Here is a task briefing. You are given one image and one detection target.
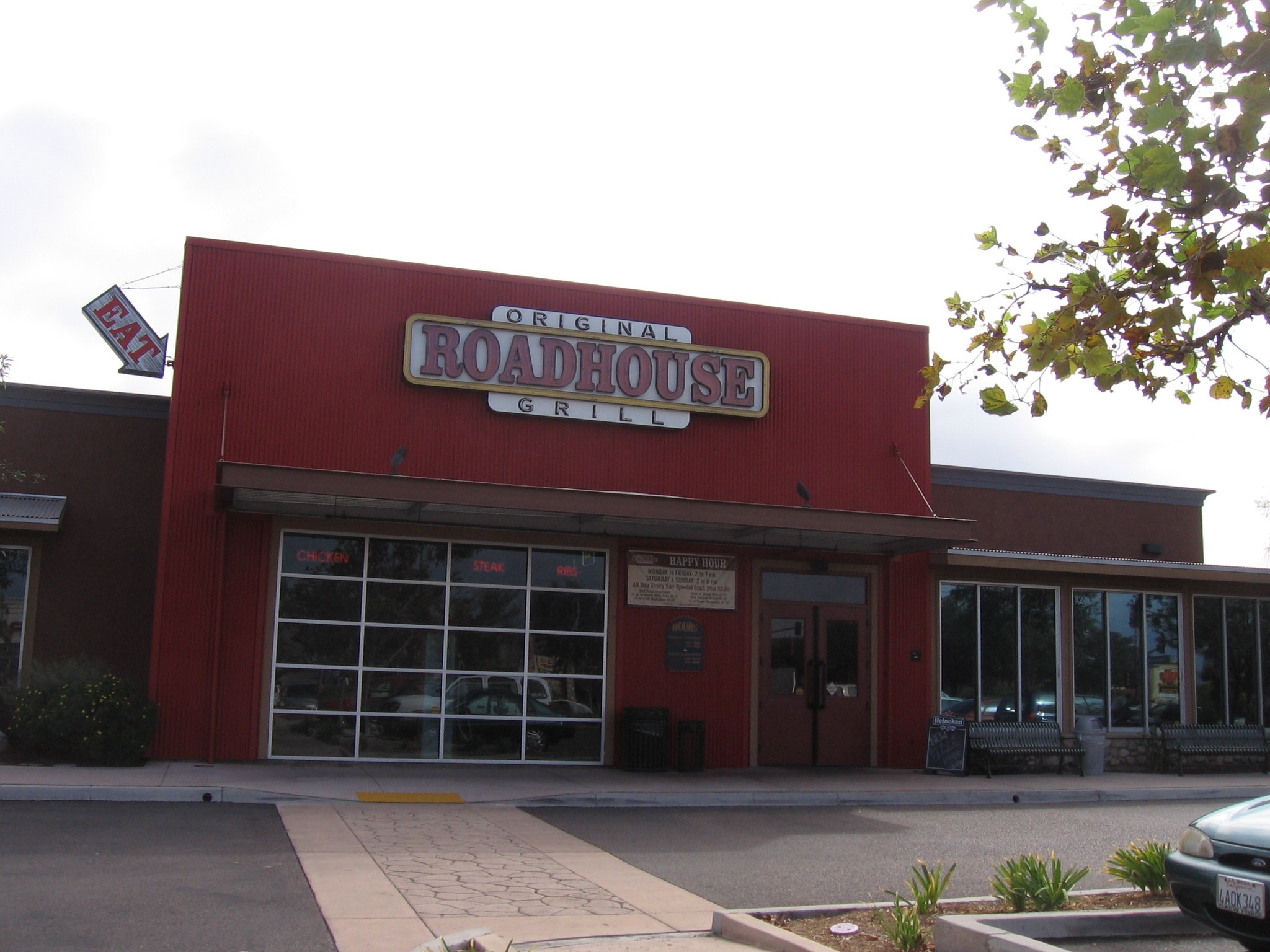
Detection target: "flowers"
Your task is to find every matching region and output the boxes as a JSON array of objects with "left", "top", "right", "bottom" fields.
[{"left": 5, "top": 674, "right": 157, "bottom": 754}]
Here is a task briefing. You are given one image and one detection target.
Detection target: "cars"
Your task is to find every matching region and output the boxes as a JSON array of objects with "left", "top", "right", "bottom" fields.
[
  {"left": 370, "top": 670, "right": 600, "bottom": 753},
  {"left": 939, "top": 687, "right": 1219, "bottom": 727},
  {"left": 828, "top": 683, "right": 856, "bottom": 696},
  {"left": 273, "top": 683, "right": 334, "bottom": 735},
  {"left": 1164, "top": 794, "right": 1270, "bottom": 952}
]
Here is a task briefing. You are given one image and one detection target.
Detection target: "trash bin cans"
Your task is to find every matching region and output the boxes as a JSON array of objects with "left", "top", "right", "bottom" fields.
[
  {"left": 1075, "top": 715, "right": 1108, "bottom": 776},
  {"left": 621, "top": 705, "right": 671, "bottom": 770}
]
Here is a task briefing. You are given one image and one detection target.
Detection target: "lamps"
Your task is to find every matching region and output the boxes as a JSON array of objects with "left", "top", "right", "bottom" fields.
[
  {"left": 796, "top": 481, "right": 811, "bottom": 507},
  {"left": 391, "top": 446, "right": 406, "bottom": 475}
]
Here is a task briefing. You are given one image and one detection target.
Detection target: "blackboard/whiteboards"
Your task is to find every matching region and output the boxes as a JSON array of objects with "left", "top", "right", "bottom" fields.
[{"left": 924, "top": 717, "right": 971, "bottom": 773}]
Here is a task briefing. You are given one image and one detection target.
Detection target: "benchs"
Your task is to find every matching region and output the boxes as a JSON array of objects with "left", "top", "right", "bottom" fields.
[
  {"left": 1159, "top": 725, "right": 1270, "bottom": 776},
  {"left": 970, "top": 722, "right": 1085, "bottom": 781}
]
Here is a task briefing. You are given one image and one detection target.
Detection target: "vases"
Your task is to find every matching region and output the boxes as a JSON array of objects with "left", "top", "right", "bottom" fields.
[
  {"left": 10, "top": 736, "right": 77, "bottom": 767},
  {"left": 77, "top": 746, "right": 150, "bottom": 769}
]
